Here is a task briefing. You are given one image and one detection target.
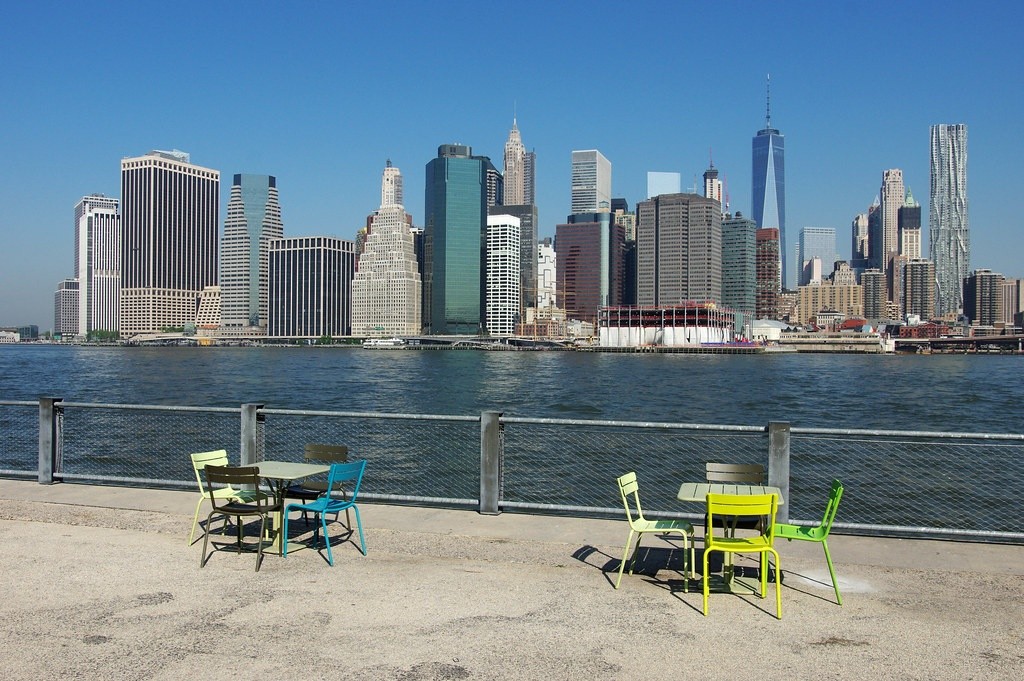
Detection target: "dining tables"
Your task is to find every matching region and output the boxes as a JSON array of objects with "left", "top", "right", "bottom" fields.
[{"left": 763, "top": 477, "right": 844, "bottom": 605}]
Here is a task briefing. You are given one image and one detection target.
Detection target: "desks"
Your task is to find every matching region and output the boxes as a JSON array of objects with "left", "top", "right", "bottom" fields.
[
  {"left": 236, "top": 460, "right": 330, "bottom": 555},
  {"left": 677, "top": 482, "right": 784, "bottom": 595}
]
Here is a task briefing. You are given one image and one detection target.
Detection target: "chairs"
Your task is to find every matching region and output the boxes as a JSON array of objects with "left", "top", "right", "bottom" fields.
[
  {"left": 702, "top": 463, "right": 768, "bottom": 576},
  {"left": 614, "top": 472, "right": 695, "bottom": 593},
  {"left": 283, "top": 459, "right": 367, "bottom": 566},
  {"left": 188, "top": 450, "right": 269, "bottom": 548},
  {"left": 201, "top": 464, "right": 283, "bottom": 572},
  {"left": 282, "top": 444, "right": 351, "bottom": 532},
  {"left": 704, "top": 493, "right": 781, "bottom": 620}
]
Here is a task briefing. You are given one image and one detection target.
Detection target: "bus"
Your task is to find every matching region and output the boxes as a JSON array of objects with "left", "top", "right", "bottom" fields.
[
  {"left": 574, "top": 337, "right": 600, "bottom": 345},
  {"left": 940, "top": 334, "right": 963, "bottom": 339}
]
